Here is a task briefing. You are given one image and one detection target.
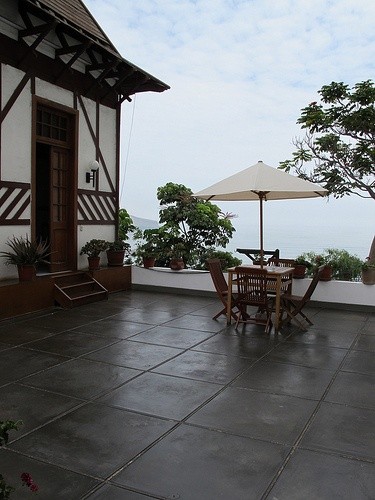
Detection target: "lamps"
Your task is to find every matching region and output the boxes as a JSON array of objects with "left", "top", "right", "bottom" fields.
[{"left": 86, "top": 160, "right": 100, "bottom": 188}]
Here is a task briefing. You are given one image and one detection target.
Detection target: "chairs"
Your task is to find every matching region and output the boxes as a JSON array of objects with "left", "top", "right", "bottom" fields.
[
  {"left": 279, "top": 262, "right": 327, "bottom": 331},
  {"left": 206, "top": 258, "right": 255, "bottom": 322},
  {"left": 253, "top": 258, "right": 295, "bottom": 321},
  {"left": 234, "top": 266, "right": 285, "bottom": 334}
]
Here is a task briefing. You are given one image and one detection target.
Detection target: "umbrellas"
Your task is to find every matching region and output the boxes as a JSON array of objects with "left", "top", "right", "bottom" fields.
[{"left": 190, "top": 161, "right": 329, "bottom": 269}]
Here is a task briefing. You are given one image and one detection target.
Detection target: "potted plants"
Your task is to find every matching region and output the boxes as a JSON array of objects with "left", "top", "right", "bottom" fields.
[
  {"left": 0, "top": 232, "right": 58, "bottom": 282},
  {"left": 292, "top": 248, "right": 375, "bottom": 285},
  {"left": 253, "top": 250, "right": 268, "bottom": 266},
  {"left": 79, "top": 239, "right": 114, "bottom": 269},
  {"left": 137, "top": 249, "right": 160, "bottom": 268},
  {"left": 106, "top": 240, "right": 132, "bottom": 266},
  {"left": 167, "top": 243, "right": 186, "bottom": 271}
]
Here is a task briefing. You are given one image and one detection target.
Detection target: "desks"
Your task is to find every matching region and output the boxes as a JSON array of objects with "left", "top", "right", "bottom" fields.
[{"left": 227, "top": 265, "right": 294, "bottom": 331}]
